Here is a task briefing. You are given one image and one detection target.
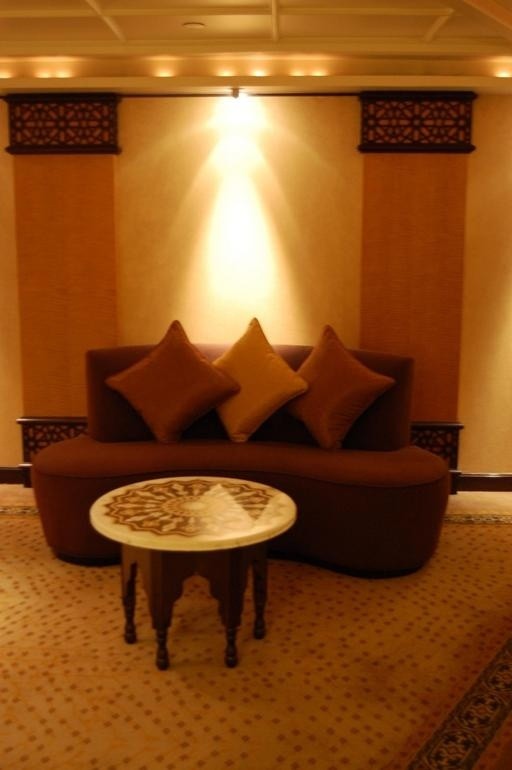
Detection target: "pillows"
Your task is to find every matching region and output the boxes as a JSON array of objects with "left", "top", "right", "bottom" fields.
[
  {"left": 107, "top": 317, "right": 240, "bottom": 444},
  {"left": 295, "top": 324, "right": 396, "bottom": 448},
  {"left": 212, "top": 317, "right": 309, "bottom": 446}
]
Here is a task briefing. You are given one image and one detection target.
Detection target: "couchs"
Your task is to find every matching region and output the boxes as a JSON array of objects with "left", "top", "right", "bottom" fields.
[{"left": 31, "top": 345, "right": 452, "bottom": 580}]
